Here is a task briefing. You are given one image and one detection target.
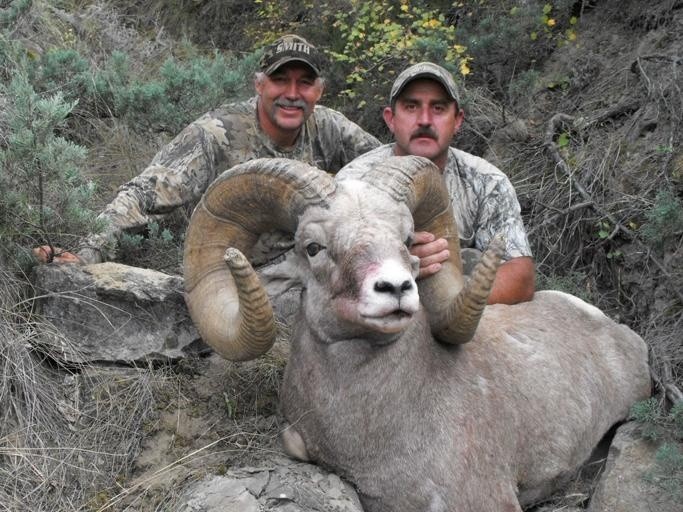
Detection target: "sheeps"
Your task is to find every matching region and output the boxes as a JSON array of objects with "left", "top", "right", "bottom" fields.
[{"left": 181, "top": 155, "right": 653, "bottom": 512}]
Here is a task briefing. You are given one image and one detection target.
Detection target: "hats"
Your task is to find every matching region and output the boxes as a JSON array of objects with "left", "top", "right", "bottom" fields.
[
  {"left": 389, "top": 61, "right": 460, "bottom": 106},
  {"left": 259, "top": 33, "right": 322, "bottom": 80}
]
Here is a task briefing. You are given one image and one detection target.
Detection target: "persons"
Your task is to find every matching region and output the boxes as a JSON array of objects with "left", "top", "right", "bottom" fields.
[
  {"left": 335, "top": 58, "right": 536, "bottom": 305},
  {"left": 33, "top": 33, "right": 382, "bottom": 266}
]
